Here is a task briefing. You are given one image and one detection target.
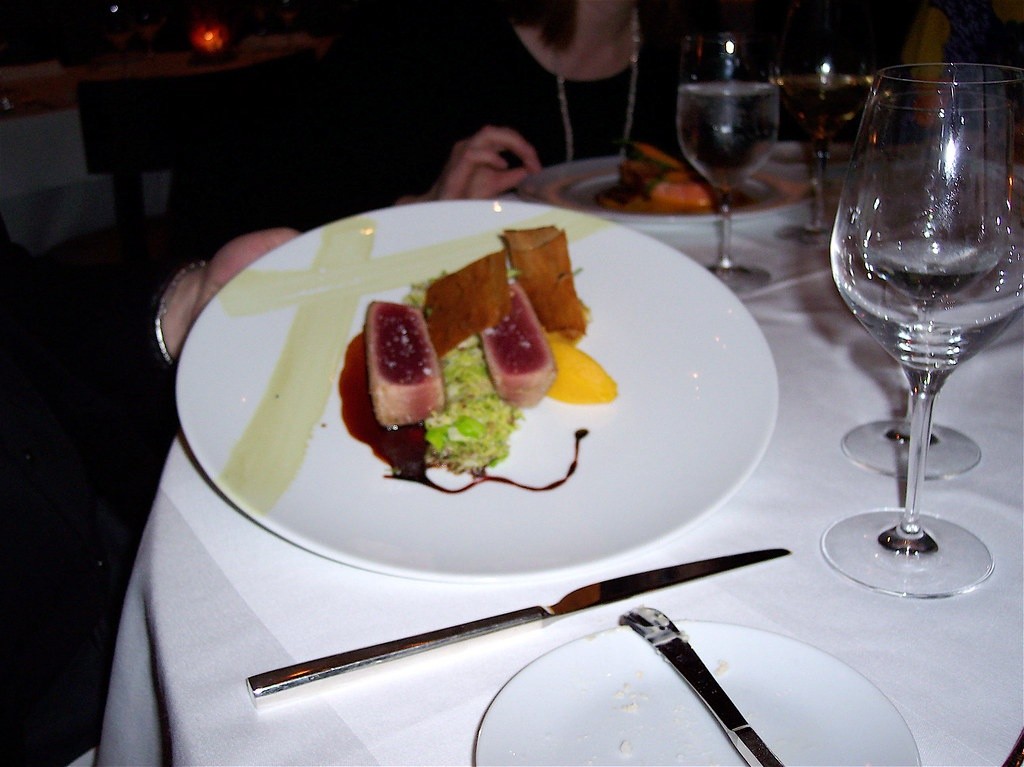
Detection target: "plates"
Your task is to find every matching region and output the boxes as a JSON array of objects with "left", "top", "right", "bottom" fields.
[
  {"left": 474, "top": 618, "right": 922, "bottom": 767},
  {"left": 517, "top": 155, "right": 814, "bottom": 258},
  {"left": 173, "top": 196, "right": 781, "bottom": 584}
]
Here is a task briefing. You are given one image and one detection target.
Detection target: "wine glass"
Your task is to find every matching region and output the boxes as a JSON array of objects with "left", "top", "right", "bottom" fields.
[
  {"left": 840, "top": 95, "right": 1024, "bottom": 482},
  {"left": 827, "top": 57, "right": 1024, "bottom": 601},
  {"left": 677, "top": 32, "right": 780, "bottom": 294}
]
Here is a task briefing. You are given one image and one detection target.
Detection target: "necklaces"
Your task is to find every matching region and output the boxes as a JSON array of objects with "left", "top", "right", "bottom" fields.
[{"left": 550, "top": 10, "right": 638, "bottom": 165}]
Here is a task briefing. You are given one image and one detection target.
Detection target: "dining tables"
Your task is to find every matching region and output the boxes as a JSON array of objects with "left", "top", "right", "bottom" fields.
[{"left": 67, "top": 140, "right": 1024, "bottom": 767}]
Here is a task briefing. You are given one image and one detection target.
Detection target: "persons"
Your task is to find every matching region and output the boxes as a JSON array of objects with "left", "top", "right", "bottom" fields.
[{"left": 0, "top": 0, "right": 1024, "bottom": 766}]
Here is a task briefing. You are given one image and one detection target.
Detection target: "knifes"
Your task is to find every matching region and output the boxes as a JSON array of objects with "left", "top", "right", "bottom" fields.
[{"left": 244, "top": 548, "right": 791, "bottom": 700}]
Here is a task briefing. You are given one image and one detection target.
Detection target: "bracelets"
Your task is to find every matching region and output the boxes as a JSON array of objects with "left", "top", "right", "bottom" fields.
[{"left": 155, "top": 256, "right": 205, "bottom": 371}]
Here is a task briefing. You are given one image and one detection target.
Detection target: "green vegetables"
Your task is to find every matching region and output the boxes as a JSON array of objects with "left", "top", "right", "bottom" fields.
[{"left": 400, "top": 266, "right": 524, "bottom": 471}]
